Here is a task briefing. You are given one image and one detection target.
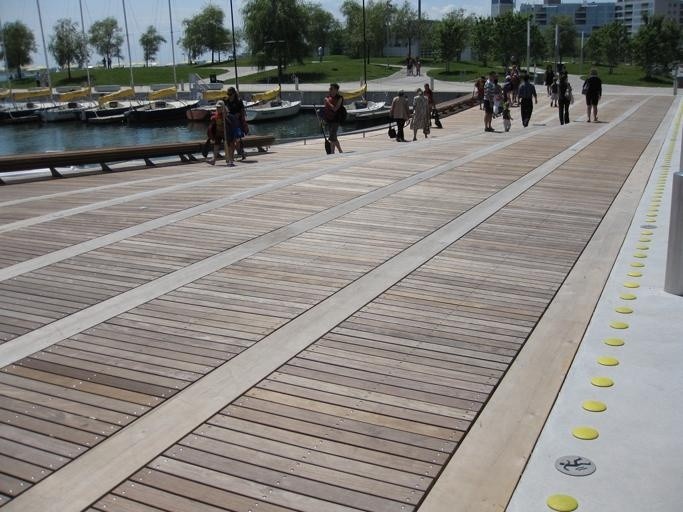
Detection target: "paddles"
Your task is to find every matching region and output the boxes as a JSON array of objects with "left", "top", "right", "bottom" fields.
[
  {"left": 203, "top": 105, "right": 245, "bottom": 164},
  {"left": 312, "top": 102, "right": 331, "bottom": 154}
]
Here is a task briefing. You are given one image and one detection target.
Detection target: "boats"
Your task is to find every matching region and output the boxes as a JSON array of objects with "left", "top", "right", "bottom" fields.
[
  {"left": 0, "top": 61, "right": 158, "bottom": 74},
  {"left": 247, "top": 119, "right": 297, "bottom": 139},
  {"left": 316, "top": 84, "right": 384, "bottom": 121},
  {"left": 123, "top": 87, "right": 199, "bottom": 125},
  {"left": 186, "top": 90, "right": 254, "bottom": 120},
  {"left": 0, "top": 89, "right": 64, "bottom": 121},
  {"left": 33, "top": 86, "right": 99, "bottom": 122},
  {"left": 236, "top": 85, "right": 301, "bottom": 122},
  {"left": 80, "top": 88, "right": 154, "bottom": 120}
]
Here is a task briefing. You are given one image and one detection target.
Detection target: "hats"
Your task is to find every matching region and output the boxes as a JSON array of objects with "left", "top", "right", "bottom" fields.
[{"left": 215, "top": 100, "right": 223, "bottom": 107}]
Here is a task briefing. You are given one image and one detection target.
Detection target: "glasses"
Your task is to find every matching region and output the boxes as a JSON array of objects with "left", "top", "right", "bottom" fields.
[{"left": 227, "top": 93, "right": 232, "bottom": 96}]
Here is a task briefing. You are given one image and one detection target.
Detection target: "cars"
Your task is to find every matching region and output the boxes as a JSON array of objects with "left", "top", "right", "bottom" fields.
[{"left": 669, "top": 64, "right": 683, "bottom": 87}]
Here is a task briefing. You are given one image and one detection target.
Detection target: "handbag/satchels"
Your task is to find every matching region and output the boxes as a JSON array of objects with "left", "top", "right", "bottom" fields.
[{"left": 388, "top": 127, "right": 396, "bottom": 139}]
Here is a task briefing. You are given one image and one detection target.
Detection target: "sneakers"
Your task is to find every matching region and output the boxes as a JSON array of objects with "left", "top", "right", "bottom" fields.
[{"left": 207, "top": 159, "right": 215, "bottom": 165}]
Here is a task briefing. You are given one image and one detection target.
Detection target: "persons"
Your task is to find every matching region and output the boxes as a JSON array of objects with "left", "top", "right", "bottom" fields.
[
  {"left": 107, "top": 56, "right": 112, "bottom": 70},
  {"left": 205, "top": 100, "right": 237, "bottom": 166},
  {"left": 414, "top": 56, "right": 422, "bottom": 77},
  {"left": 405, "top": 52, "right": 415, "bottom": 76},
  {"left": 317, "top": 46, "right": 323, "bottom": 63},
  {"left": 471, "top": 55, "right": 573, "bottom": 132},
  {"left": 582, "top": 69, "right": 602, "bottom": 122},
  {"left": 35, "top": 71, "right": 41, "bottom": 86},
  {"left": 390, "top": 90, "right": 409, "bottom": 142},
  {"left": 323, "top": 82, "right": 344, "bottom": 154},
  {"left": 102, "top": 57, "right": 107, "bottom": 68},
  {"left": 42, "top": 69, "right": 51, "bottom": 87},
  {"left": 410, "top": 87, "right": 431, "bottom": 142},
  {"left": 423, "top": 83, "right": 434, "bottom": 129},
  {"left": 223, "top": 87, "right": 249, "bottom": 162}
]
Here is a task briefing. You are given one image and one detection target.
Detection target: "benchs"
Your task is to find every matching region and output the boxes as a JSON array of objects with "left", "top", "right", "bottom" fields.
[
  {"left": 432, "top": 90, "right": 478, "bottom": 119},
  {"left": 1, "top": 133, "right": 274, "bottom": 183}
]
